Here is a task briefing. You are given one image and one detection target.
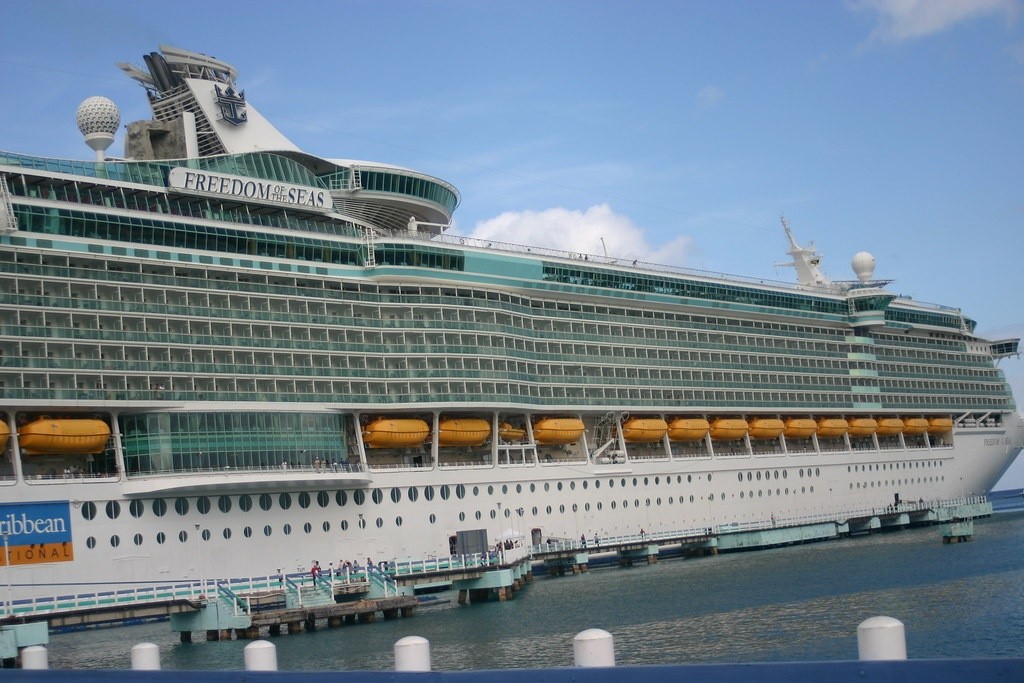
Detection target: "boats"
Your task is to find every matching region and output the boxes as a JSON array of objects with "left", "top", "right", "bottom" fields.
[
  {"left": 362, "top": 419, "right": 429, "bottom": 450},
  {"left": 439, "top": 419, "right": 490, "bottom": 447},
  {"left": 498, "top": 428, "right": 525, "bottom": 440},
  {"left": 621, "top": 417, "right": 953, "bottom": 441},
  {"left": 17, "top": 419, "right": 111, "bottom": 456},
  {"left": 533, "top": 418, "right": 585, "bottom": 444}
]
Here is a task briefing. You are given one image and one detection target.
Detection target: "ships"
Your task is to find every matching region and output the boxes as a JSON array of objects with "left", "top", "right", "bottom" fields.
[{"left": 0, "top": 47, "right": 1024, "bottom": 635}]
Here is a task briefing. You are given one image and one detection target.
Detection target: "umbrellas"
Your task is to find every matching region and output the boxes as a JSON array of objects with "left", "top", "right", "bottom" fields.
[{"left": 495, "top": 528, "right": 526, "bottom": 541}]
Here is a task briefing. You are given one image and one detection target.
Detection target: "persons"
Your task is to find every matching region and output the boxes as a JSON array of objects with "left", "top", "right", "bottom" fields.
[
  {"left": 314, "top": 456, "right": 350, "bottom": 474},
  {"left": 354, "top": 459, "right": 360, "bottom": 470},
  {"left": 337, "top": 559, "right": 360, "bottom": 576},
  {"left": 310, "top": 561, "right": 322, "bottom": 586},
  {"left": 281, "top": 460, "right": 287, "bottom": 473},
  {"left": 329, "top": 561, "right": 334, "bottom": 577},
  {"left": 771, "top": 514, "right": 776, "bottom": 526},
  {"left": 851, "top": 437, "right": 871, "bottom": 450},
  {"left": 494, "top": 540, "right": 524, "bottom": 558},
  {"left": 63, "top": 467, "right": 70, "bottom": 479},
  {"left": 581, "top": 534, "right": 586, "bottom": 548},
  {"left": 366, "top": 557, "right": 373, "bottom": 573},
  {"left": 640, "top": 528, "right": 646, "bottom": 541},
  {"left": 75, "top": 467, "right": 83, "bottom": 478},
  {"left": 919, "top": 496, "right": 923, "bottom": 509},
  {"left": 594, "top": 533, "right": 600, "bottom": 547}
]
